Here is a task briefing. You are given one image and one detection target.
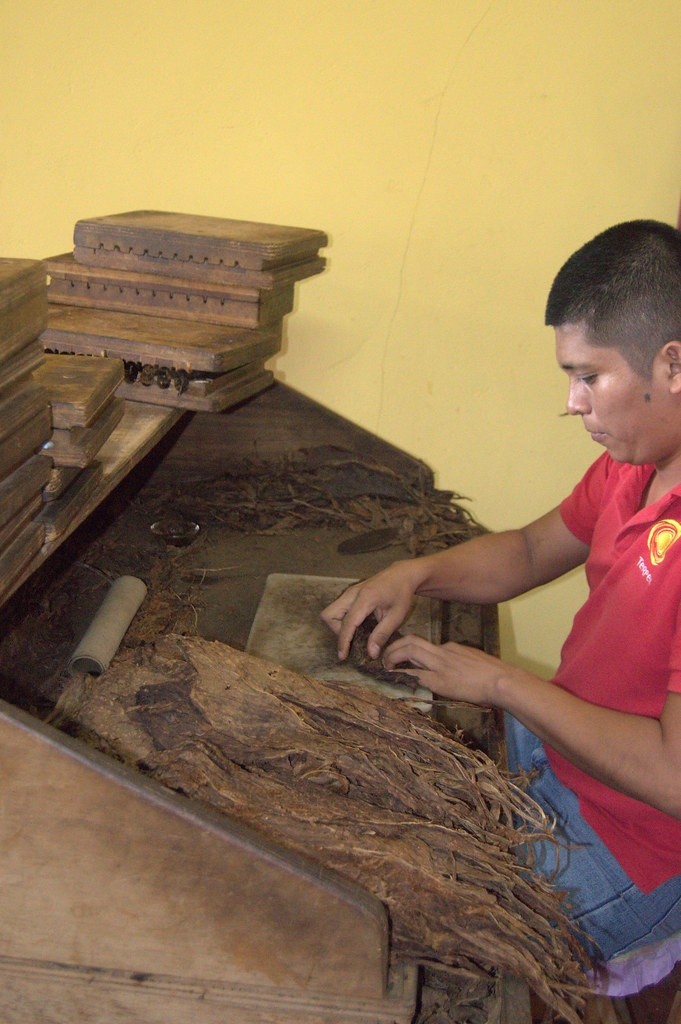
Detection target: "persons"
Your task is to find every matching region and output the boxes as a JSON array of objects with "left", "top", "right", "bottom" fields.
[{"left": 321, "top": 220, "right": 681, "bottom": 973}]
